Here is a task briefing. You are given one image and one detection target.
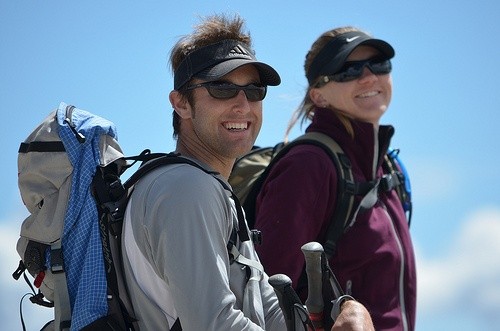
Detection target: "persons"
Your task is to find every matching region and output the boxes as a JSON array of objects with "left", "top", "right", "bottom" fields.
[
  {"left": 117, "top": 9, "right": 377, "bottom": 331},
  {"left": 250, "top": 24, "right": 418, "bottom": 331}
]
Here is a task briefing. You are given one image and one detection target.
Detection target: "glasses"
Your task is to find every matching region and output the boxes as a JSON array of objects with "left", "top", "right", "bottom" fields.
[
  {"left": 188, "top": 79, "right": 267, "bottom": 102},
  {"left": 311, "top": 54, "right": 392, "bottom": 89}
]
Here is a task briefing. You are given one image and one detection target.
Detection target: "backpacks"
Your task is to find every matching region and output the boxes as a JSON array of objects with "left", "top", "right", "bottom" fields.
[
  {"left": 227, "top": 131, "right": 414, "bottom": 300},
  {"left": 12, "top": 100, "right": 266, "bottom": 331}
]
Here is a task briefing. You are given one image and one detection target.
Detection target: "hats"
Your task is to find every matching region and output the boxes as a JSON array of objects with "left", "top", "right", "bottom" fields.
[
  {"left": 305, "top": 30, "right": 395, "bottom": 86},
  {"left": 173, "top": 38, "right": 281, "bottom": 90}
]
